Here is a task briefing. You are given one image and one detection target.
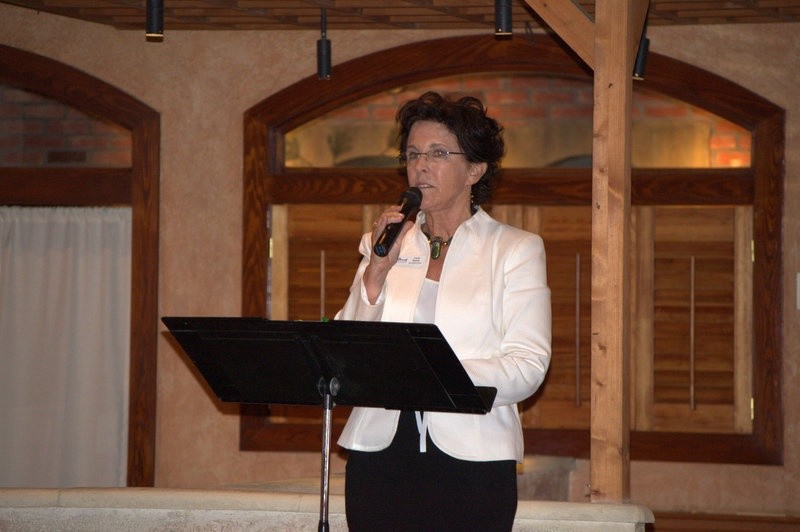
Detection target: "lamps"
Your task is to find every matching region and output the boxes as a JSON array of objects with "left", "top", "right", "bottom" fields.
[
  {"left": 146, "top": 0, "right": 163, "bottom": 41},
  {"left": 493, "top": 0, "right": 512, "bottom": 36},
  {"left": 632, "top": 0, "right": 651, "bottom": 80},
  {"left": 317, "top": 8, "right": 331, "bottom": 80}
]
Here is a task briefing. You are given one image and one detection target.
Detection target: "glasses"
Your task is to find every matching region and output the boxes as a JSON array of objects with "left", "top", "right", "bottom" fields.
[{"left": 400, "top": 145, "right": 467, "bottom": 163}]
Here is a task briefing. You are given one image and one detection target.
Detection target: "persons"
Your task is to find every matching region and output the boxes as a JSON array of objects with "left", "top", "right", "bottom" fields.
[{"left": 333, "top": 91, "right": 551, "bottom": 532}]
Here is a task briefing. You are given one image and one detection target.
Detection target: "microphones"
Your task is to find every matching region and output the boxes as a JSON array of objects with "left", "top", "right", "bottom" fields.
[{"left": 373, "top": 186, "right": 424, "bottom": 258}]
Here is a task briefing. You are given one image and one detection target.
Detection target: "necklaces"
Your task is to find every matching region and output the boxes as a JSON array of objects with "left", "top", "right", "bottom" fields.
[{"left": 422, "top": 229, "right": 454, "bottom": 259}]
